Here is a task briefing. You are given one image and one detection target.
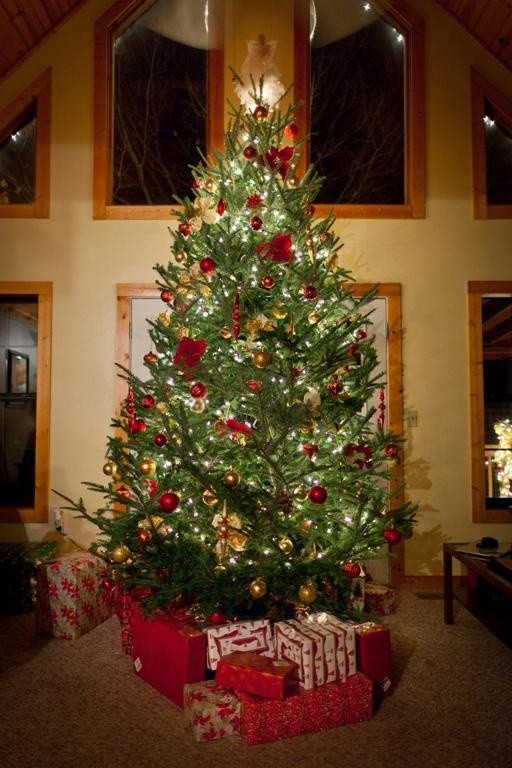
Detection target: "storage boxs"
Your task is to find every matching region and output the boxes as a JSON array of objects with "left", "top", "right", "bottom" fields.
[
  {"left": 36, "top": 550, "right": 115, "bottom": 641},
  {"left": 120, "top": 581, "right": 395, "bottom": 746}
]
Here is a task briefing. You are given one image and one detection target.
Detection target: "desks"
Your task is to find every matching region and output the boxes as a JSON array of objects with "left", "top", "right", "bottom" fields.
[{"left": 442, "top": 541, "right": 512, "bottom": 653}]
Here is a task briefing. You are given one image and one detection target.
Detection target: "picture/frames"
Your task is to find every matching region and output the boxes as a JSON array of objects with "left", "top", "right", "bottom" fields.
[{"left": 5, "top": 349, "right": 29, "bottom": 408}]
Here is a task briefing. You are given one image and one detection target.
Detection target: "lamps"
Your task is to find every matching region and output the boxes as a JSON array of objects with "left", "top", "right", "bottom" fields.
[{"left": 235, "top": 32, "right": 287, "bottom": 115}]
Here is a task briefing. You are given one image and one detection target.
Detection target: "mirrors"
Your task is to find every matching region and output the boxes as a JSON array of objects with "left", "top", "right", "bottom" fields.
[{"left": 0, "top": 281, "right": 53, "bottom": 525}]
